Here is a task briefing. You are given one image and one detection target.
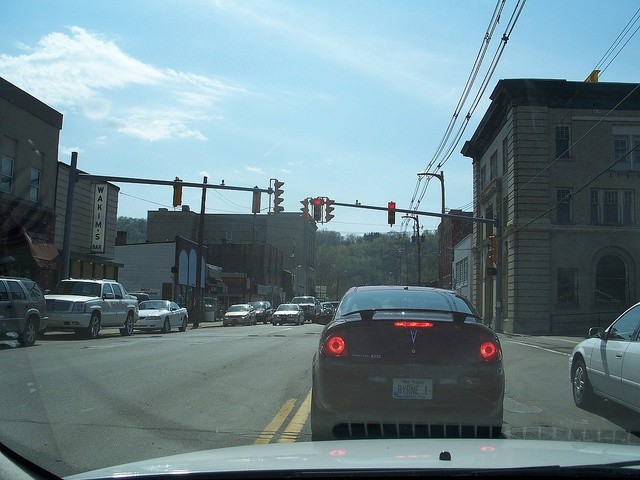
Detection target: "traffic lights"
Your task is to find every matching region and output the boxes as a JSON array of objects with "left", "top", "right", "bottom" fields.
[
  {"left": 323, "top": 197, "right": 334, "bottom": 223},
  {"left": 310, "top": 199, "right": 323, "bottom": 222},
  {"left": 489, "top": 236, "right": 498, "bottom": 263},
  {"left": 269, "top": 179, "right": 284, "bottom": 215},
  {"left": 300, "top": 197, "right": 312, "bottom": 223},
  {"left": 387, "top": 202, "right": 395, "bottom": 224}
]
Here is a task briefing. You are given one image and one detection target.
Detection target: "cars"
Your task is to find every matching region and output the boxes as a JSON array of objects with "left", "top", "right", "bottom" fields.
[
  {"left": 569, "top": 303, "right": 639, "bottom": 412},
  {"left": 317, "top": 302, "right": 335, "bottom": 323},
  {"left": 272, "top": 303, "right": 304, "bottom": 325},
  {"left": 248, "top": 301, "right": 273, "bottom": 324},
  {"left": 133, "top": 300, "right": 188, "bottom": 334},
  {"left": 224, "top": 303, "right": 256, "bottom": 325},
  {"left": 313, "top": 286, "right": 504, "bottom": 434}
]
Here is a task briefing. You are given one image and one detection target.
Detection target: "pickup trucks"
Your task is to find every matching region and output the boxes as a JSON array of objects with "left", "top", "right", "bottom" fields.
[{"left": 44, "top": 280, "right": 138, "bottom": 338}]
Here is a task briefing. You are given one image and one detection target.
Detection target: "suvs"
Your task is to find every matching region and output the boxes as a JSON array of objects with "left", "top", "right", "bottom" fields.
[
  {"left": 129, "top": 291, "right": 149, "bottom": 304},
  {"left": 0, "top": 276, "right": 48, "bottom": 347},
  {"left": 291, "top": 296, "right": 321, "bottom": 323}
]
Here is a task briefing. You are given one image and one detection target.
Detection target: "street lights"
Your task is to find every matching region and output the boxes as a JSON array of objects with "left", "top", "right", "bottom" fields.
[
  {"left": 418, "top": 172, "right": 445, "bottom": 289},
  {"left": 402, "top": 216, "right": 421, "bottom": 285}
]
